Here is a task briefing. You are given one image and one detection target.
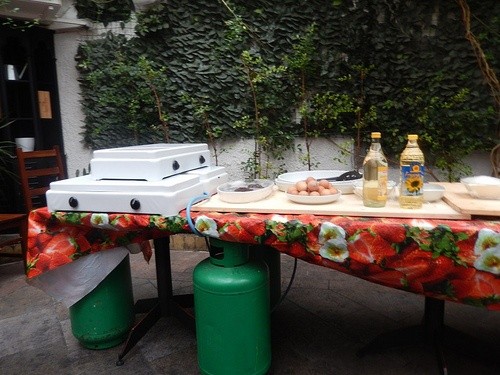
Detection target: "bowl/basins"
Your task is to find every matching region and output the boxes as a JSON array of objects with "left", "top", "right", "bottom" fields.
[
  {"left": 460, "top": 175, "right": 500, "bottom": 200},
  {"left": 15, "top": 137, "right": 35, "bottom": 152},
  {"left": 351, "top": 179, "right": 396, "bottom": 200},
  {"left": 422, "top": 183, "right": 446, "bottom": 202}
]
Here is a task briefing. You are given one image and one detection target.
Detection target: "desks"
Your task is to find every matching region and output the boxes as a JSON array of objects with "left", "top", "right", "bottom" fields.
[{"left": 27, "top": 179, "right": 500, "bottom": 375}]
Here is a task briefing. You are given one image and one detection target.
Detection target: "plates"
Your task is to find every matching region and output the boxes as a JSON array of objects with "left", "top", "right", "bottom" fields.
[
  {"left": 285, "top": 191, "right": 341, "bottom": 204},
  {"left": 217, "top": 179, "right": 274, "bottom": 204},
  {"left": 275, "top": 170, "right": 364, "bottom": 195}
]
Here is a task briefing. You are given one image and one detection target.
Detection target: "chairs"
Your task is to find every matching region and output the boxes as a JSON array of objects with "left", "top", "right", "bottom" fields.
[
  {"left": 15, "top": 144, "right": 66, "bottom": 213},
  {"left": 0, "top": 213, "right": 29, "bottom": 273}
]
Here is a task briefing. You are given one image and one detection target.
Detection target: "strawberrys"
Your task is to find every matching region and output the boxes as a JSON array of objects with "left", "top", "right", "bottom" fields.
[{"left": 26, "top": 209, "right": 500, "bottom": 309}]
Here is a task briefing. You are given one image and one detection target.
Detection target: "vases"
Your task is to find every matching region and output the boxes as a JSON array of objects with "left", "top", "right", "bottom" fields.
[{"left": 15, "top": 137, "right": 35, "bottom": 153}]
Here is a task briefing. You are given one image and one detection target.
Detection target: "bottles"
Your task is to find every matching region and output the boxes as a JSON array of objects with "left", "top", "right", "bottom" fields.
[
  {"left": 363, "top": 132, "right": 388, "bottom": 208},
  {"left": 398, "top": 135, "right": 424, "bottom": 209},
  {"left": 216, "top": 177, "right": 223, "bottom": 194}
]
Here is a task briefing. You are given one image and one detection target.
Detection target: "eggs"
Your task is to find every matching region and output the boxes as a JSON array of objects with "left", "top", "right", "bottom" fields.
[{"left": 287, "top": 177, "right": 337, "bottom": 196}]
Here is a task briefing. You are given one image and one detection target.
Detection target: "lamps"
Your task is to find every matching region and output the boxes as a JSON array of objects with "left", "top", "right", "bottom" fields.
[{"left": 4, "top": 62, "right": 28, "bottom": 81}]
